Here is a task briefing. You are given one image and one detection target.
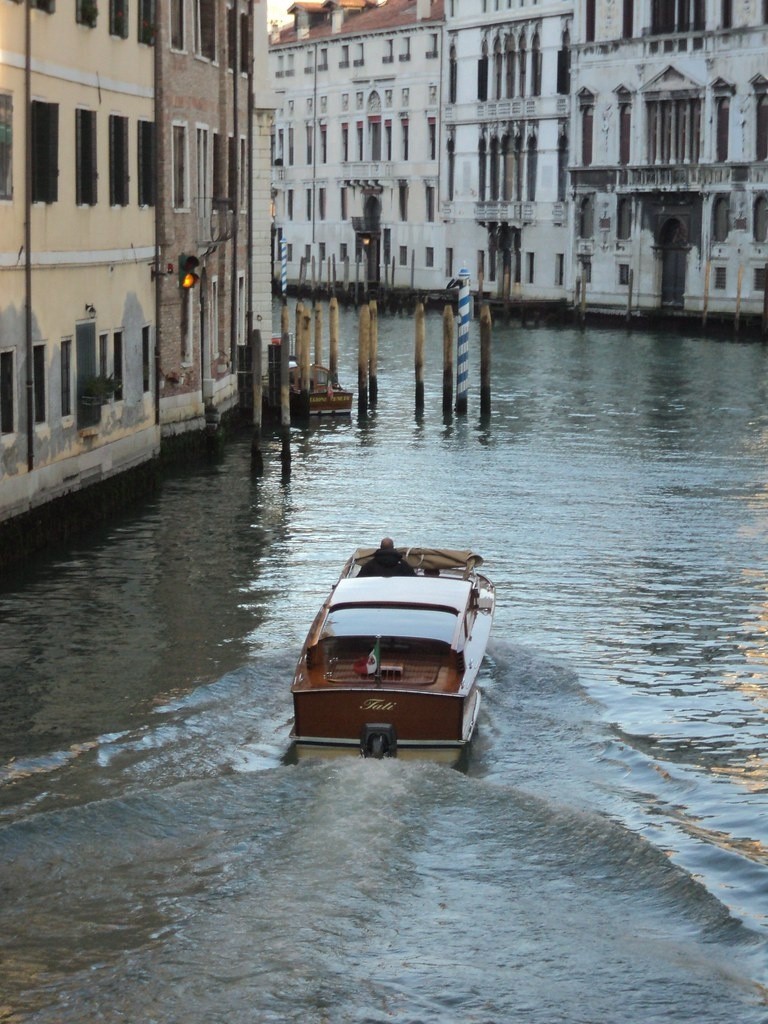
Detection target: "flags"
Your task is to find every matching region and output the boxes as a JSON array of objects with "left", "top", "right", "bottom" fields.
[{"left": 354, "top": 642, "right": 378, "bottom": 674}]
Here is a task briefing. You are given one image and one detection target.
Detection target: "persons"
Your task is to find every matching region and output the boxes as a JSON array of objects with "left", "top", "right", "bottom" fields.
[{"left": 356, "top": 538, "right": 418, "bottom": 577}]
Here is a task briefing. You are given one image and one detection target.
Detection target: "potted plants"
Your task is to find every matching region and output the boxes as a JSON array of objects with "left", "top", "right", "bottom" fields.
[{"left": 80, "top": 372, "right": 123, "bottom": 406}]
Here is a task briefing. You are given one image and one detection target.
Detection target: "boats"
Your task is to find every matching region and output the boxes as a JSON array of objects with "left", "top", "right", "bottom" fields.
[
  {"left": 286, "top": 361, "right": 353, "bottom": 415},
  {"left": 287, "top": 546, "right": 497, "bottom": 770}
]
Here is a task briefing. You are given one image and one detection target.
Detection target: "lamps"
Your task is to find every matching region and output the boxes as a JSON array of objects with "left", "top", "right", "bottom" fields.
[{"left": 85, "top": 303, "right": 96, "bottom": 319}]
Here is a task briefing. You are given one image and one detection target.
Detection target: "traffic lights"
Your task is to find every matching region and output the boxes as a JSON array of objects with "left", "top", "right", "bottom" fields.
[{"left": 178, "top": 254, "right": 201, "bottom": 290}]
